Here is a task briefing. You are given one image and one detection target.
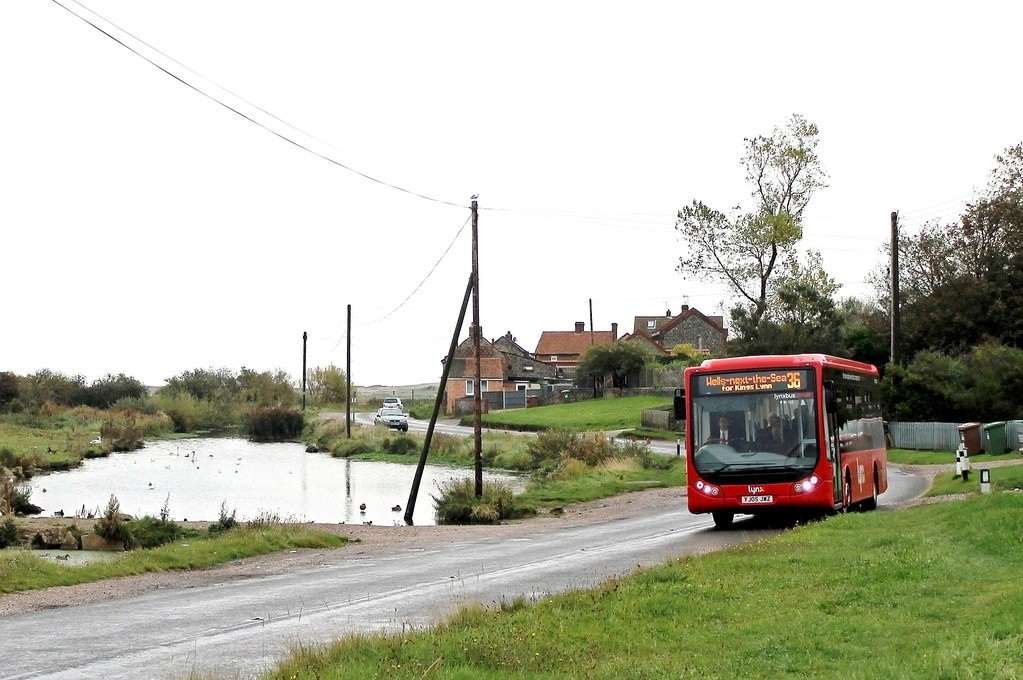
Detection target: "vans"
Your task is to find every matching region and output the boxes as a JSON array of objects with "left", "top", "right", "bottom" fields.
[{"left": 382, "top": 398, "right": 402, "bottom": 409}]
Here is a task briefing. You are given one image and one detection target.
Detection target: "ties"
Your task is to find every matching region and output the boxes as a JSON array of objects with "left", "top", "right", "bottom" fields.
[{"left": 722, "top": 432, "right": 725, "bottom": 444}]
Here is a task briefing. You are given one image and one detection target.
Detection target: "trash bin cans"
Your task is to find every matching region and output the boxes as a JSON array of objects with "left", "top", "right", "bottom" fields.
[
  {"left": 982, "top": 420, "right": 1011, "bottom": 457},
  {"left": 957, "top": 422, "right": 982, "bottom": 458}
]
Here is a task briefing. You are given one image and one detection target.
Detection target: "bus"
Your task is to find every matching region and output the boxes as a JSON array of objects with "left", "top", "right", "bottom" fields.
[{"left": 671, "top": 352, "right": 890, "bottom": 530}]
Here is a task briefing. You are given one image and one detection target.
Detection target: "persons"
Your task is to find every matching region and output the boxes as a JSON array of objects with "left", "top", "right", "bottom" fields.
[
  {"left": 707, "top": 416, "right": 735, "bottom": 446},
  {"left": 754, "top": 405, "right": 813, "bottom": 449}
]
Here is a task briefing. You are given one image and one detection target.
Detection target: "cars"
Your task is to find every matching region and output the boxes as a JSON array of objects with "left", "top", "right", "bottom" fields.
[{"left": 374, "top": 407, "right": 409, "bottom": 432}]
